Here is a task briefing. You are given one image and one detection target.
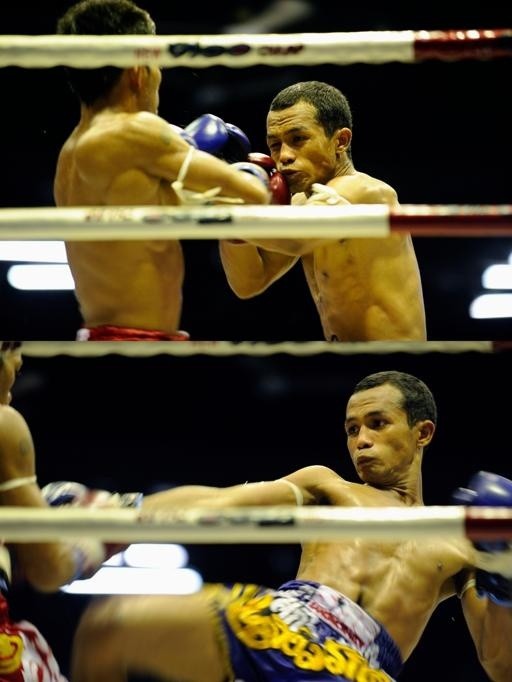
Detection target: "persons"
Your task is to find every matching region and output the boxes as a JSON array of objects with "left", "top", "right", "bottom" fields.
[
  {"left": 41, "top": 372, "right": 512, "bottom": 682},
  {"left": 54, "top": 1, "right": 269, "bottom": 341},
  {"left": 219, "top": 81, "right": 428, "bottom": 340},
  {"left": 0, "top": 341, "right": 131, "bottom": 682}
]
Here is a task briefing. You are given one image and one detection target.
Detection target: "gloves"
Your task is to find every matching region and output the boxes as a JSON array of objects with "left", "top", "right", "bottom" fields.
[
  {"left": 42, "top": 481, "right": 143, "bottom": 586},
  {"left": 171, "top": 113, "right": 252, "bottom": 162},
  {"left": 453, "top": 468, "right": 512, "bottom": 609},
  {"left": 231, "top": 152, "right": 290, "bottom": 206}
]
